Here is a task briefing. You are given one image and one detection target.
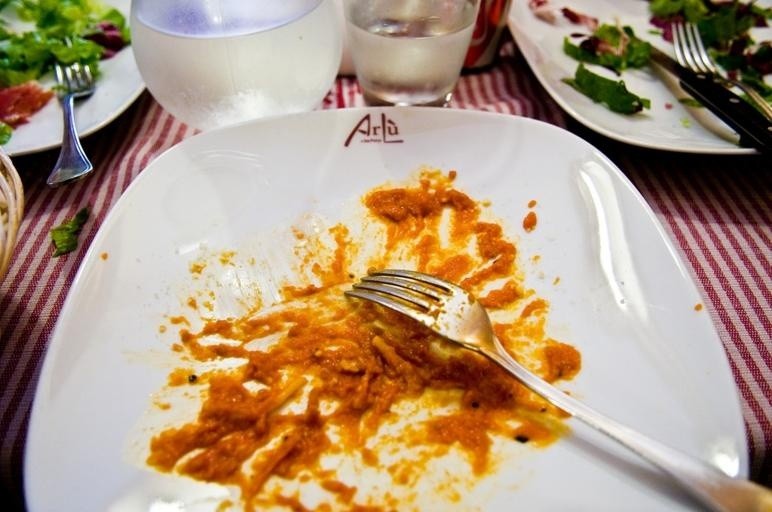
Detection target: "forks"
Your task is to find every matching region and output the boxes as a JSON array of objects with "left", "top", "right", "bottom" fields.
[
  {"left": 672, "top": 16, "right": 772, "bottom": 124},
  {"left": 342, "top": 266, "right": 771, "bottom": 509},
  {"left": 44, "top": 61, "right": 102, "bottom": 185}
]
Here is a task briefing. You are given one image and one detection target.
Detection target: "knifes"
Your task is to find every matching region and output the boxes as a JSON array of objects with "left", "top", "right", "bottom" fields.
[{"left": 571, "top": 10, "right": 770, "bottom": 157}]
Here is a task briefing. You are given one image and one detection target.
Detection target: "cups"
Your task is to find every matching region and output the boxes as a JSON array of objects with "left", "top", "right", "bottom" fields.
[
  {"left": 344, "top": 2, "right": 480, "bottom": 108},
  {"left": 133, "top": 0, "right": 346, "bottom": 125}
]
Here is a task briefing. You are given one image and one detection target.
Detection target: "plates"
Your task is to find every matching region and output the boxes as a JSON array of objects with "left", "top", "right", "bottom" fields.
[
  {"left": 508, "top": 1, "right": 763, "bottom": 155},
  {"left": 0, "top": 0, "right": 146, "bottom": 154},
  {"left": 21, "top": 104, "right": 751, "bottom": 505}
]
